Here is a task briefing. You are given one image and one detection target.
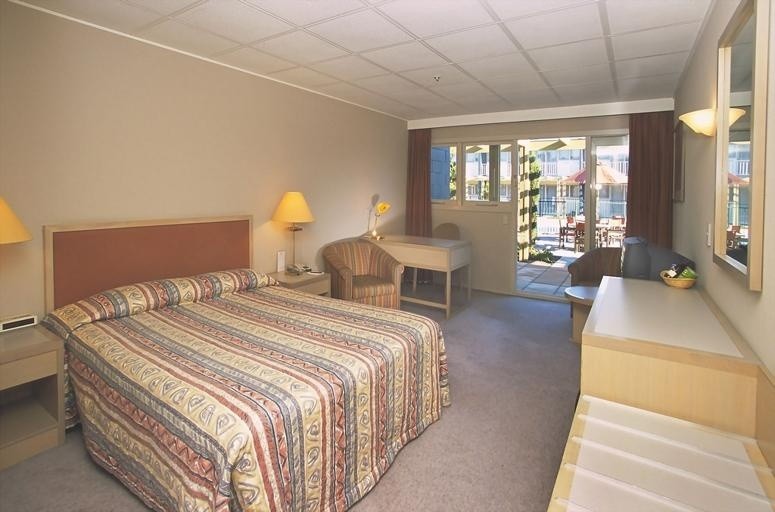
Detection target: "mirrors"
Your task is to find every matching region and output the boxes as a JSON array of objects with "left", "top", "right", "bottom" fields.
[{"left": 713, "top": 0, "right": 771, "bottom": 295}]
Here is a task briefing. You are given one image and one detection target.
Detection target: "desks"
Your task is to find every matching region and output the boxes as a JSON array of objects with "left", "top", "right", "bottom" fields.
[{"left": 379, "top": 233, "right": 471, "bottom": 316}]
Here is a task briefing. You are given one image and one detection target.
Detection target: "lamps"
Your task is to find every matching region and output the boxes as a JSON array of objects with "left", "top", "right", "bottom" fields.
[
  {"left": 0, "top": 199, "right": 34, "bottom": 244},
  {"left": 272, "top": 190, "right": 314, "bottom": 272},
  {"left": 676, "top": 105, "right": 713, "bottom": 136},
  {"left": 729, "top": 106, "right": 747, "bottom": 132},
  {"left": 370, "top": 202, "right": 390, "bottom": 236}
]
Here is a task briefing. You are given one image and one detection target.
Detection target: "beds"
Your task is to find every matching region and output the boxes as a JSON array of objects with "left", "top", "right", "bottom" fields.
[{"left": 40, "top": 216, "right": 452, "bottom": 512}]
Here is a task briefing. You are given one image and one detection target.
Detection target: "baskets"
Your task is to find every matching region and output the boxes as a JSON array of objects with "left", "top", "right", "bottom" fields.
[{"left": 659, "top": 269, "right": 697, "bottom": 289}]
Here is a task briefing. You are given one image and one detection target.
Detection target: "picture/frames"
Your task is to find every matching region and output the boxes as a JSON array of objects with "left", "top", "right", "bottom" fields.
[{"left": 672, "top": 115, "right": 686, "bottom": 202}]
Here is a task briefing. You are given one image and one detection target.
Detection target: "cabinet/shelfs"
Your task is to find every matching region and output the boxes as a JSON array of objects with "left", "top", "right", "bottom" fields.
[
  {"left": 578, "top": 277, "right": 759, "bottom": 432},
  {"left": 0, "top": 322, "right": 64, "bottom": 472},
  {"left": 266, "top": 268, "right": 330, "bottom": 298},
  {"left": 550, "top": 395, "right": 774, "bottom": 512}
]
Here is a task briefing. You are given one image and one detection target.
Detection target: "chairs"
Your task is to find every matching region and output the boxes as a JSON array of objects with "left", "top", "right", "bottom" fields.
[
  {"left": 322, "top": 237, "right": 403, "bottom": 310},
  {"left": 430, "top": 225, "right": 461, "bottom": 294},
  {"left": 557, "top": 207, "right": 626, "bottom": 252}
]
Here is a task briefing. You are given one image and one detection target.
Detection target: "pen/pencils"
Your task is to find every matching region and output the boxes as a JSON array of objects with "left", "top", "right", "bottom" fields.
[{"left": 311, "top": 272, "right": 322, "bottom": 273}]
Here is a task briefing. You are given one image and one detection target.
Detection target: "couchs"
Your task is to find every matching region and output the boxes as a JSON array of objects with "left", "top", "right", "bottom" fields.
[{"left": 567, "top": 245, "right": 697, "bottom": 319}]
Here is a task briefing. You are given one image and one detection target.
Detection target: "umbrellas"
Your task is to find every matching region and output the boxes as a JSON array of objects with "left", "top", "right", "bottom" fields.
[
  {"left": 557, "top": 160, "right": 629, "bottom": 223},
  {"left": 727, "top": 171, "right": 749, "bottom": 187}
]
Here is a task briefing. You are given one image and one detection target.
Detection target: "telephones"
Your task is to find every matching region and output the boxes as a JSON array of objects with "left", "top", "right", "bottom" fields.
[{"left": 287, "top": 264, "right": 306, "bottom": 273}]
